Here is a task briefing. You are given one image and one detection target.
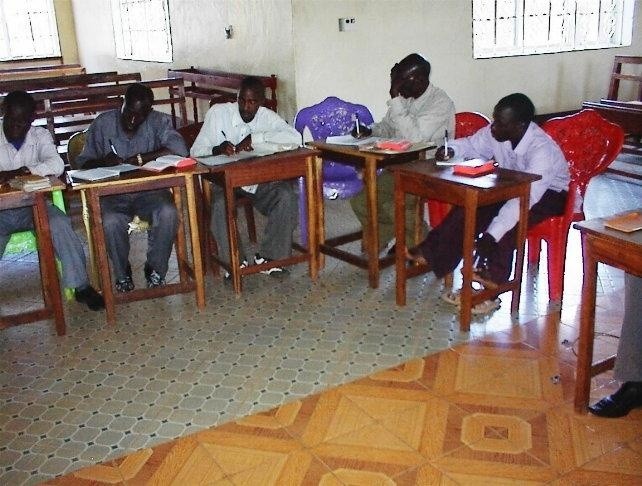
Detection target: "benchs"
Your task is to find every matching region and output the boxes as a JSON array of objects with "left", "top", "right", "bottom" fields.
[
  {"left": 0, "top": 71, "right": 142, "bottom": 95},
  {"left": 581, "top": 56, "right": 642, "bottom": 180},
  {"left": 0, "top": 63, "right": 86, "bottom": 81},
  {"left": 0, "top": 56, "right": 64, "bottom": 71},
  {"left": 0, "top": 79, "right": 187, "bottom": 168}
]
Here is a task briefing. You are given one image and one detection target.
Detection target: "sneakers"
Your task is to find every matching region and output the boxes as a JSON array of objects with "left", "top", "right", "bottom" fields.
[
  {"left": 113, "top": 263, "right": 134, "bottom": 292},
  {"left": 145, "top": 262, "right": 165, "bottom": 289},
  {"left": 376, "top": 235, "right": 397, "bottom": 260},
  {"left": 253, "top": 253, "right": 282, "bottom": 277},
  {"left": 74, "top": 286, "right": 106, "bottom": 313},
  {"left": 224, "top": 260, "right": 249, "bottom": 278}
]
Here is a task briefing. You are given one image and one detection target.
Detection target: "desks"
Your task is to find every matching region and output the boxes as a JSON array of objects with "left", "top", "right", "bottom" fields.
[
  {"left": 387, "top": 158, "right": 541, "bottom": 332},
  {"left": 573, "top": 209, "right": 641, "bottom": 414},
  {"left": 196, "top": 147, "right": 324, "bottom": 292},
  {"left": 305, "top": 137, "right": 425, "bottom": 288},
  {"left": 0, "top": 177, "right": 67, "bottom": 336},
  {"left": 71, "top": 158, "right": 210, "bottom": 326}
]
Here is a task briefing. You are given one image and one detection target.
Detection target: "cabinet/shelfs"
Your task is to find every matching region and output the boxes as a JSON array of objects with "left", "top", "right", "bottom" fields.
[{"left": 165, "top": 66, "right": 278, "bottom": 127}]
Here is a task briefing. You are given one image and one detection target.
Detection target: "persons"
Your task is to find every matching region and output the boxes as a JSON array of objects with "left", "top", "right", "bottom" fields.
[
  {"left": 73, "top": 84, "right": 189, "bottom": 294},
  {"left": 0, "top": 90, "right": 106, "bottom": 311},
  {"left": 350, "top": 53, "right": 456, "bottom": 261},
  {"left": 403, "top": 93, "right": 572, "bottom": 315},
  {"left": 189, "top": 77, "right": 303, "bottom": 283},
  {"left": 588, "top": 272, "right": 642, "bottom": 418}
]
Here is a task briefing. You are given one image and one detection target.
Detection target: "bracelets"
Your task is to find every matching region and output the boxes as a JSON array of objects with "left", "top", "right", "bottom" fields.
[{"left": 136, "top": 153, "right": 143, "bottom": 167}]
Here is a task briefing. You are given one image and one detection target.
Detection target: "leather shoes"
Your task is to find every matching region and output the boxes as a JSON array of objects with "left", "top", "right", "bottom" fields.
[{"left": 587, "top": 380, "right": 641, "bottom": 418}]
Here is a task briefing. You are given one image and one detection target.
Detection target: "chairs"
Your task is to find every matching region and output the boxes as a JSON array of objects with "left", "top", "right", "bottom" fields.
[
  {"left": 428, "top": 111, "right": 491, "bottom": 230},
  {"left": 67, "top": 130, "right": 175, "bottom": 292},
  {"left": 175, "top": 124, "right": 258, "bottom": 279},
  {"left": 294, "top": 97, "right": 376, "bottom": 248},
  {"left": 526, "top": 109, "right": 625, "bottom": 301},
  {"left": 4, "top": 190, "right": 77, "bottom": 300}
]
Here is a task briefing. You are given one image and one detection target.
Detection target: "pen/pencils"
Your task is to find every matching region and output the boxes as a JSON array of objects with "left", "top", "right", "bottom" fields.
[
  {"left": 221, "top": 130, "right": 237, "bottom": 153},
  {"left": 445, "top": 129, "right": 448, "bottom": 160},
  {"left": 355, "top": 114, "right": 361, "bottom": 139},
  {"left": 109, "top": 138, "right": 123, "bottom": 165}
]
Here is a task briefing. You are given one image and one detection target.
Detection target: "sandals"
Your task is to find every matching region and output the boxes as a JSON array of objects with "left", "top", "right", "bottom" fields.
[
  {"left": 463, "top": 297, "right": 503, "bottom": 315},
  {"left": 440, "top": 288, "right": 472, "bottom": 304}
]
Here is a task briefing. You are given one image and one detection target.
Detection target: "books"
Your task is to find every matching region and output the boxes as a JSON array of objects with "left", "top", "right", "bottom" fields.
[
  {"left": 71, "top": 163, "right": 139, "bottom": 181},
  {"left": 604, "top": 210, "right": 642, "bottom": 232},
  {"left": 141, "top": 154, "right": 186, "bottom": 172},
  {"left": 326, "top": 134, "right": 390, "bottom": 146},
  {"left": 454, "top": 164, "right": 495, "bottom": 177},
  {"left": 435, "top": 159, "right": 486, "bottom": 167},
  {"left": 9, "top": 174, "right": 52, "bottom": 193},
  {"left": 377, "top": 140, "right": 412, "bottom": 150}
]
